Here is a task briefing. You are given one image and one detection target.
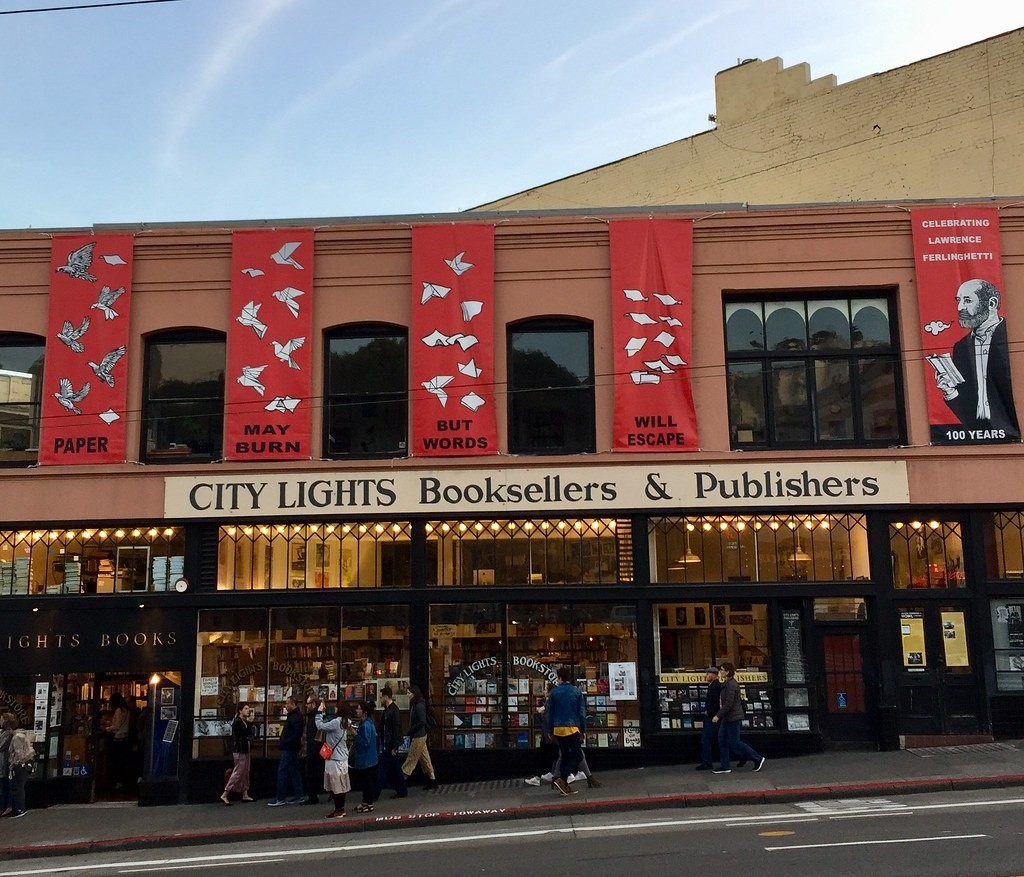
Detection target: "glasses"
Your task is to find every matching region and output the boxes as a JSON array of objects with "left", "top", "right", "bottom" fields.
[
  {"left": 720, "top": 669, "right": 723, "bottom": 671},
  {"left": 305, "top": 701, "right": 313, "bottom": 705}
]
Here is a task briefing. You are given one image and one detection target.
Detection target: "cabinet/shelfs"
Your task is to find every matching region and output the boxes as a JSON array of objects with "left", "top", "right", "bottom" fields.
[
  {"left": 65, "top": 735, "right": 110, "bottom": 803},
  {"left": 220, "top": 635, "right": 623, "bottom": 752},
  {"left": 74, "top": 671, "right": 170, "bottom": 731},
  {"left": 658, "top": 682, "right": 774, "bottom": 730}
]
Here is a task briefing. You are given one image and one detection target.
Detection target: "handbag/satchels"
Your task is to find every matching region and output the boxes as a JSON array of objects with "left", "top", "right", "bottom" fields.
[
  {"left": 741, "top": 698, "right": 748, "bottom": 715},
  {"left": 319, "top": 743, "right": 333, "bottom": 760}
]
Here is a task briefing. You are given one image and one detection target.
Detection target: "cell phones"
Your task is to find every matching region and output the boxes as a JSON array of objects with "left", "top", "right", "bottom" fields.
[{"left": 322, "top": 698, "right": 324, "bottom": 702}]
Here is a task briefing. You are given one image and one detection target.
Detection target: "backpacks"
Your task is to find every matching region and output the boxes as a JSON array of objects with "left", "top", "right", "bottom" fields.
[{"left": 412, "top": 698, "right": 438, "bottom": 733}]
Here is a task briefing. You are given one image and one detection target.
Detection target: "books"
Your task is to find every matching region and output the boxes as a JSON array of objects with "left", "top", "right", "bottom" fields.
[
  {"left": 444, "top": 640, "right": 530, "bottom": 749},
  {"left": 530, "top": 637, "right": 609, "bottom": 680},
  {"left": 271, "top": 643, "right": 338, "bottom": 685},
  {"left": 532, "top": 679, "right": 641, "bottom": 747},
  {"left": 219, "top": 647, "right": 238, "bottom": 702},
  {"left": 340, "top": 660, "right": 399, "bottom": 702},
  {"left": 0, "top": 556, "right": 183, "bottom": 595},
  {"left": 238, "top": 684, "right": 338, "bottom": 702},
  {"left": 658, "top": 685, "right": 707, "bottom": 728},
  {"left": 740, "top": 685, "right": 774, "bottom": 728}
]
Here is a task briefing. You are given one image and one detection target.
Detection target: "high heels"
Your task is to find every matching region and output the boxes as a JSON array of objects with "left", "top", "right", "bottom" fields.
[
  {"left": 551, "top": 775, "right": 560, "bottom": 790},
  {"left": 586, "top": 775, "right": 603, "bottom": 788}
]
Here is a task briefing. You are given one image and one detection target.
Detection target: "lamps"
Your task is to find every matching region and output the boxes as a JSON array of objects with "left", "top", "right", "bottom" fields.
[
  {"left": 787, "top": 520, "right": 811, "bottom": 560},
  {"left": 677, "top": 521, "right": 701, "bottom": 563}
]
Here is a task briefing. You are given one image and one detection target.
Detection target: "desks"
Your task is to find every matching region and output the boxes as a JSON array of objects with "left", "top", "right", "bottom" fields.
[{"left": 97, "top": 576, "right": 135, "bottom": 592}]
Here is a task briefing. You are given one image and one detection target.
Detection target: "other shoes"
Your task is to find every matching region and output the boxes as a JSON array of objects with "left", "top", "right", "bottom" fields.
[
  {"left": 422, "top": 784, "right": 438, "bottom": 790},
  {"left": 0, "top": 807, "right": 13, "bottom": 817},
  {"left": 390, "top": 792, "right": 408, "bottom": 799},
  {"left": 299, "top": 796, "right": 319, "bottom": 805},
  {"left": 241, "top": 797, "right": 257, "bottom": 802},
  {"left": 219, "top": 796, "right": 234, "bottom": 806}
]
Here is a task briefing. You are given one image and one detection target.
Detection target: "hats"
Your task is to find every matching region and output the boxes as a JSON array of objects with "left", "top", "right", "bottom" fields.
[{"left": 705, "top": 667, "right": 719, "bottom": 676}]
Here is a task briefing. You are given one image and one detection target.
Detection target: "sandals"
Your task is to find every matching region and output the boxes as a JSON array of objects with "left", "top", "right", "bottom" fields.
[{"left": 351, "top": 803, "right": 374, "bottom": 813}]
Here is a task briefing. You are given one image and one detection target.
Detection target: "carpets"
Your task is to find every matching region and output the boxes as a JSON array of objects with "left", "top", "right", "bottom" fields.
[
  {"left": 47, "top": 803, "right": 134, "bottom": 808},
  {"left": 905, "top": 741, "right": 1020, "bottom": 758}
]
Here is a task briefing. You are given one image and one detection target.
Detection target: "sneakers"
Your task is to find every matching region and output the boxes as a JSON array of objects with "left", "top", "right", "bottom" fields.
[
  {"left": 574, "top": 771, "right": 587, "bottom": 780},
  {"left": 267, "top": 798, "right": 288, "bottom": 807},
  {"left": 736, "top": 754, "right": 749, "bottom": 767},
  {"left": 541, "top": 772, "right": 554, "bottom": 782},
  {"left": 566, "top": 773, "right": 576, "bottom": 784},
  {"left": 286, "top": 797, "right": 306, "bottom": 804},
  {"left": 525, "top": 777, "right": 541, "bottom": 787},
  {"left": 710, "top": 763, "right": 732, "bottom": 774},
  {"left": 553, "top": 777, "right": 570, "bottom": 796},
  {"left": 8, "top": 809, "right": 28, "bottom": 819},
  {"left": 752, "top": 756, "right": 766, "bottom": 772},
  {"left": 325, "top": 808, "right": 347, "bottom": 819},
  {"left": 559, "top": 783, "right": 579, "bottom": 796},
  {"left": 695, "top": 763, "right": 714, "bottom": 771}
]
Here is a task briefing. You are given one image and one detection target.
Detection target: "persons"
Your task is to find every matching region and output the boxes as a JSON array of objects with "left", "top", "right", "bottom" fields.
[
  {"left": 124, "top": 696, "right": 143, "bottom": 788},
  {"left": 376, "top": 684, "right": 439, "bottom": 799},
  {"left": 567, "top": 771, "right": 586, "bottom": 784},
  {"left": 220, "top": 702, "right": 258, "bottom": 806},
  {"left": 695, "top": 662, "right": 765, "bottom": 774},
  {"left": 0, "top": 713, "right": 37, "bottom": 818},
  {"left": 299, "top": 695, "right": 334, "bottom": 805},
  {"left": 100, "top": 692, "right": 130, "bottom": 789},
  {"left": 676, "top": 609, "right": 687, "bottom": 626},
  {"left": 349, "top": 702, "right": 377, "bottom": 813},
  {"left": 268, "top": 696, "right": 304, "bottom": 806},
  {"left": 551, "top": 683, "right": 605, "bottom": 790},
  {"left": 315, "top": 698, "right": 352, "bottom": 819},
  {"left": 524, "top": 683, "right": 558, "bottom": 787},
  {"left": 546, "top": 668, "right": 587, "bottom": 795}
]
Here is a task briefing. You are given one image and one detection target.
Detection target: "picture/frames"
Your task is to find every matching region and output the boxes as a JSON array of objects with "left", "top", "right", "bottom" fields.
[
  {"left": 326, "top": 628, "right": 338, "bottom": 637},
  {"left": 233, "top": 631, "right": 241, "bottom": 642},
  {"left": 368, "top": 626, "right": 382, "bottom": 638},
  {"left": 282, "top": 629, "right": 297, "bottom": 640},
  {"left": 244, "top": 631, "right": 259, "bottom": 642},
  {"left": 564, "top": 623, "right": 586, "bottom": 634},
  {"left": 473, "top": 623, "right": 496, "bottom": 634},
  {"left": 347, "top": 626, "right": 362, "bottom": 630},
  {"left": 303, "top": 628, "right": 322, "bottom": 637},
  {"left": 160, "top": 687, "right": 180, "bottom": 743},
  {"left": 261, "top": 630, "right": 276, "bottom": 640}
]
